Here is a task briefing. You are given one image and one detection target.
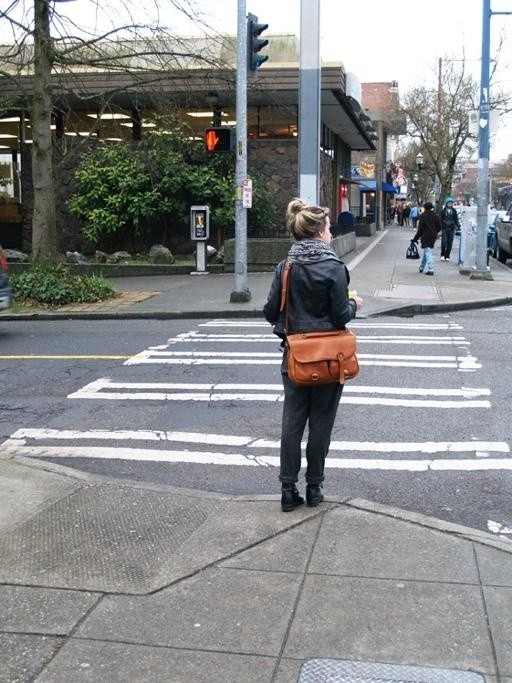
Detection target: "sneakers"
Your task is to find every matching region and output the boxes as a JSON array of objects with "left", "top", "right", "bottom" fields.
[
  {"left": 419, "top": 267, "right": 433, "bottom": 275},
  {"left": 440, "top": 255, "right": 450, "bottom": 261}
]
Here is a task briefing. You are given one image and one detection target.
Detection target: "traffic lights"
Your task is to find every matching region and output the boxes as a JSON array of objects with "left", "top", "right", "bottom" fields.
[
  {"left": 246, "top": 11, "right": 269, "bottom": 78},
  {"left": 204, "top": 126, "right": 232, "bottom": 154}
]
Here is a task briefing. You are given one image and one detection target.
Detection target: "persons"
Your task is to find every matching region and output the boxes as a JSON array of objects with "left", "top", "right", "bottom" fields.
[
  {"left": 409, "top": 201, "right": 442, "bottom": 276},
  {"left": 386, "top": 202, "right": 425, "bottom": 233},
  {"left": 261, "top": 197, "right": 367, "bottom": 514},
  {"left": 439, "top": 196, "right": 461, "bottom": 263}
]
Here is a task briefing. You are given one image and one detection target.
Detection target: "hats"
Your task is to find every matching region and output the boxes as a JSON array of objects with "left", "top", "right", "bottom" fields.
[{"left": 445, "top": 197, "right": 454, "bottom": 206}]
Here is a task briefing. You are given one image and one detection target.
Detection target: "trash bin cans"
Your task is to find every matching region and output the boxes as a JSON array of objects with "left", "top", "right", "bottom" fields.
[{"left": 457, "top": 225, "right": 496, "bottom": 267}]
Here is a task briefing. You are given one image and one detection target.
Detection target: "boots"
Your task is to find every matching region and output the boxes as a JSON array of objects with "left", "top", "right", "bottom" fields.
[
  {"left": 281, "top": 483, "right": 303, "bottom": 512},
  {"left": 306, "top": 485, "right": 323, "bottom": 508}
]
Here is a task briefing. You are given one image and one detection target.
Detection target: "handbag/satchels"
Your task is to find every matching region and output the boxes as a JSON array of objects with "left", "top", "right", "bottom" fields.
[
  {"left": 406, "top": 244, "right": 419, "bottom": 258},
  {"left": 284, "top": 328, "right": 359, "bottom": 385}
]
